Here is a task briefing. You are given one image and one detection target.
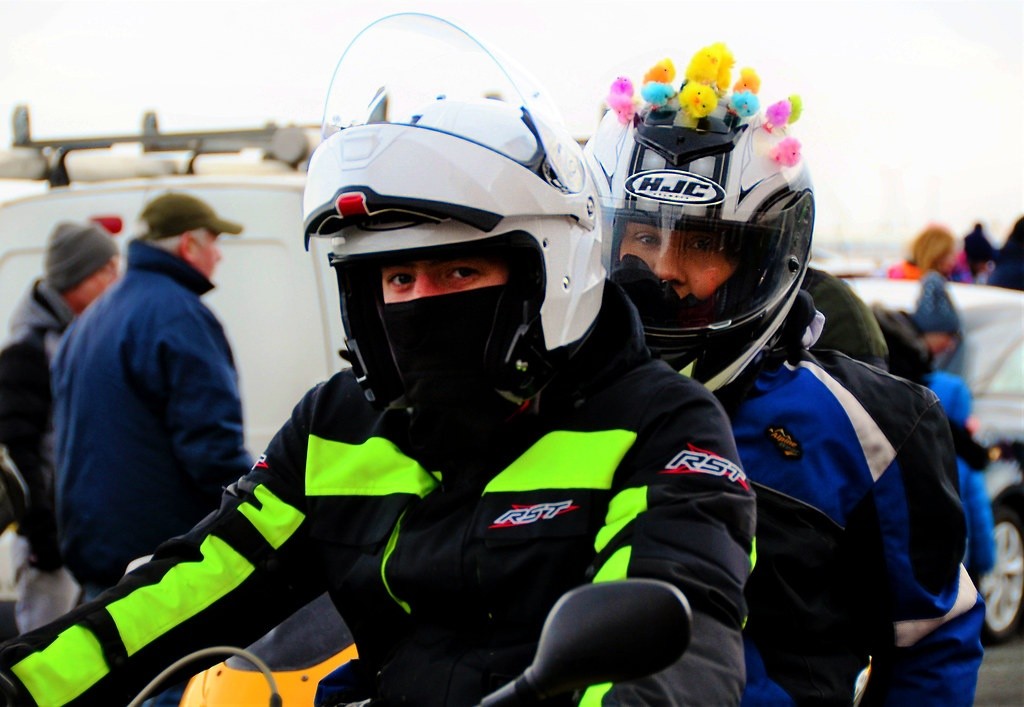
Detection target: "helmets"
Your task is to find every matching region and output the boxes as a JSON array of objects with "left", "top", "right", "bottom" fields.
[
  {"left": 583, "top": 95, "right": 817, "bottom": 399},
  {"left": 300, "top": 93, "right": 612, "bottom": 361}
]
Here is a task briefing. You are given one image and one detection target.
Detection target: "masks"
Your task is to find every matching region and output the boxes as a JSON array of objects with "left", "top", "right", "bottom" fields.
[{"left": 376, "top": 282, "right": 526, "bottom": 380}]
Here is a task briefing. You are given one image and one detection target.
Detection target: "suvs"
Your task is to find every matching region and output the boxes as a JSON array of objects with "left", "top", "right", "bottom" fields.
[{"left": 0, "top": 105, "right": 1024, "bottom": 644}]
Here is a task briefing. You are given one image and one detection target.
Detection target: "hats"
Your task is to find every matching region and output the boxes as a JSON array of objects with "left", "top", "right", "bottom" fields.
[
  {"left": 915, "top": 269, "right": 963, "bottom": 334},
  {"left": 136, "top": 190, "right": 244, "bottom": 241},
  {"left": 45, "top": 218, "right": 121, "bottom": 292}
]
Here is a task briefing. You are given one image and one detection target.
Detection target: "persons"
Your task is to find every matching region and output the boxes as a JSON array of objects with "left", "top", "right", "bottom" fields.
[
  {"left": 50, "top": 189, "right": 253, "bottom": 606},
  {"left": 0, "top": 95, "right": 756, "bottom": 706},
  {"left": 581, "top": 80, "right": 985, "bottom": 707},
  {"left": 874, "top": 215, "right": 1023, "bottom": 591},
  {"left": 0, "top": 219, "right": 121, "bottom": 635}
]
